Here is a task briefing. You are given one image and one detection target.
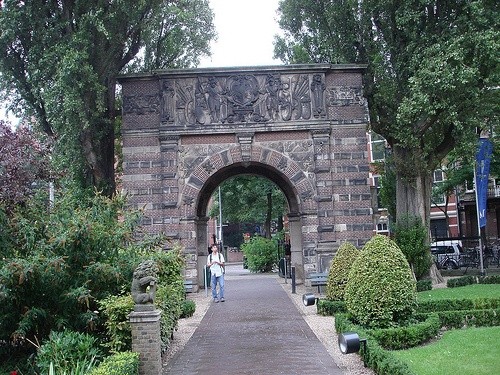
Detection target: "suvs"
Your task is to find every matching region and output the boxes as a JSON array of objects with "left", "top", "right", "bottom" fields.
[{"left": 430, "top": 240, "right": 465, "bottom": 270}]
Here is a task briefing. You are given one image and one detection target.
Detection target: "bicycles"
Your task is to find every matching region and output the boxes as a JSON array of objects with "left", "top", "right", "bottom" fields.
[{"left": 438, "top": 240, "right": 500, "bottom": 270}]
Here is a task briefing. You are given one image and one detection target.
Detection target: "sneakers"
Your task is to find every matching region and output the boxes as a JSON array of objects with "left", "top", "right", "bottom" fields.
[
  {"left": 212, "top": 297, "right": 218, "bottom": 303},
  {"left": 220, "top": 297, "right": 225, "bottom": 302}
]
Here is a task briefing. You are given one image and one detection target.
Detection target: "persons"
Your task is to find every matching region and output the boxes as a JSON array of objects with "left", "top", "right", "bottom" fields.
[{"left": 207, "top": 244, "right": 226, "bottom": 303}]
